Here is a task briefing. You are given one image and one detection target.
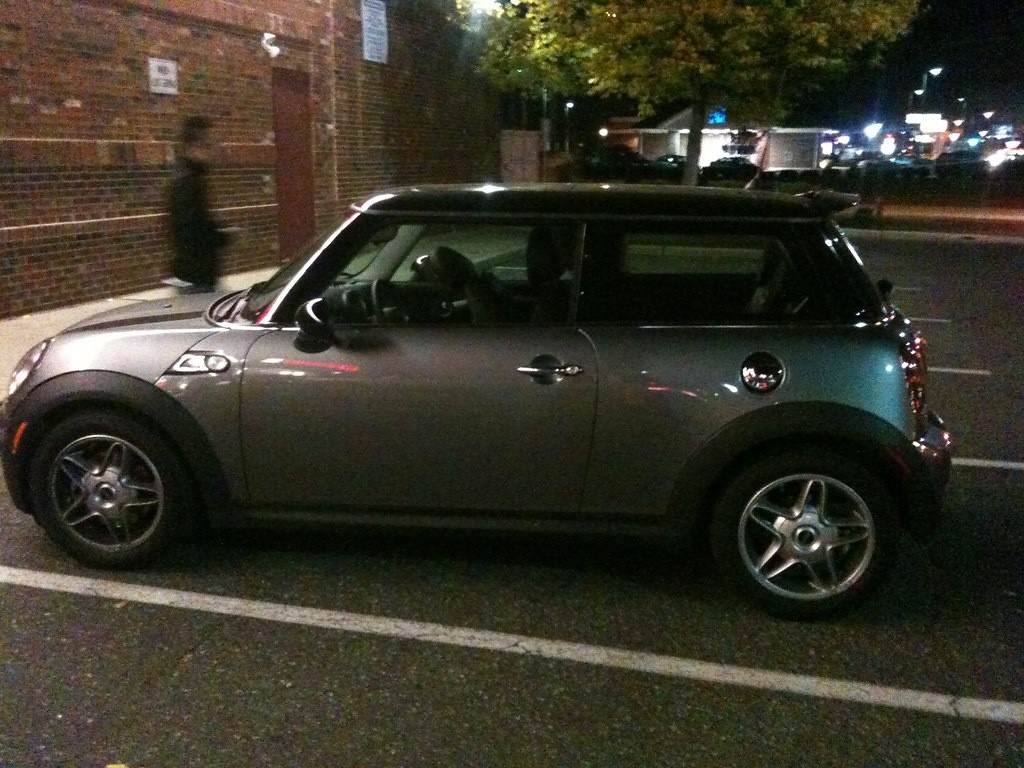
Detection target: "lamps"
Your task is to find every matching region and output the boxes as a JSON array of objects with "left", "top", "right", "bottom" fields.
[{"left": 262, "top": 32, "right": 280, "bottom": 59}]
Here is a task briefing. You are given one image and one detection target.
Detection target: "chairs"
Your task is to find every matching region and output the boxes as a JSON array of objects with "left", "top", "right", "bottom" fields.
[{"left": 503, "top": 226, "right": 636, "bottom": 323}]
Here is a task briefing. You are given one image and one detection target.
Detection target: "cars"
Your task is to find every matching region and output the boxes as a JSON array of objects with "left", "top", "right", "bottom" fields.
[
  {"left": 0, "top": 181, "right": 951, "bottom": 624},
  {"left": 705, "top": 155, "right": 762, "bottom": 175},
  {"left": 654, "top": 154, "right": 686, "bottom": 171}
]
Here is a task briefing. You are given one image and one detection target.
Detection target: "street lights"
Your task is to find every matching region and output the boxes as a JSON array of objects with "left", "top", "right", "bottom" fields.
[{"left": 908, "top": 67, "right": 943, "bottom": 111}]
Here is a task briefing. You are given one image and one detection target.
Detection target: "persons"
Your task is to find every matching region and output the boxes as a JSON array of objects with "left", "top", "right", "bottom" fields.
[
  {"left": 166, "top": 116, "right": 240, "bottom": 294},
  {"left": 467, "top": 226, "right": 572, "bottom": 324}
]
